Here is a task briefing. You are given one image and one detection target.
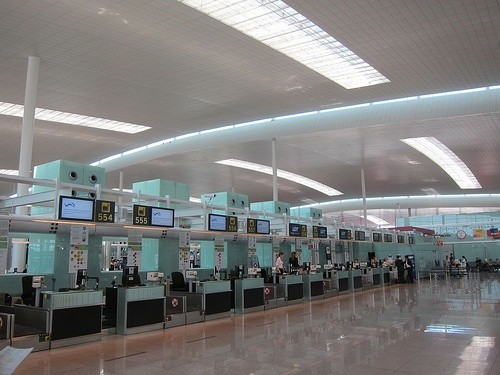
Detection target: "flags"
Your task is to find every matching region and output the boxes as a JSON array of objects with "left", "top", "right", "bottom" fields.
[{"left": 473, "top": 226, "right": 500, "bottom": 240}]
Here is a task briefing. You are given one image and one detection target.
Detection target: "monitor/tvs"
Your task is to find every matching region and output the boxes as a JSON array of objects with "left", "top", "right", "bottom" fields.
[
  {"left": 318, "top": 226, "right": 327, "bottom": 238},
  {"left": 339, "top": 228, "right": 412, "bottom": 244},
  {"left": 150, "top": 206, "right": 174, "bottom": 227},
  {"left": 208, "top": 214, "right": 228, "bottom": 231},
  {"left": 289, "top": 223, "right": 301, "bottom": 236},
  {"left": 256, "top": 218, "right": 270, "bottom": 234},
  {"left": 58, "top": 196, "right": 95, "bottom": 222}
]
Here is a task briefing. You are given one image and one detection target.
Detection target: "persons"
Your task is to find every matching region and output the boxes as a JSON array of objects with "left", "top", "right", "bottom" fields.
[
  {"left": 382, "top": 257, "right": 395, "bottom": 269},
  {"left": 288, "top": 250, "right": 301, "bottom": 274},
  {"left": 444, "top": 252, "right": 470, "bottom": 274},
  {"left": 402, "top": 256, "right": 415, "bottom": 285},
  {"left": 275, "top": 251, "right": 284, "bottom": 277},
  {"left": 395, "top": 254, "right": 407, "bottom": 284},
  {"left": 353, "top": 258, "right": 358, "bottom": 269}
]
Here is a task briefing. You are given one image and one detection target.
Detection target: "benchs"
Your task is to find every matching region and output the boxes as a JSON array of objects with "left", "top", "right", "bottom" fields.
[{"left": 419, "top": 261, "right": 500, "bottom": 282}]
